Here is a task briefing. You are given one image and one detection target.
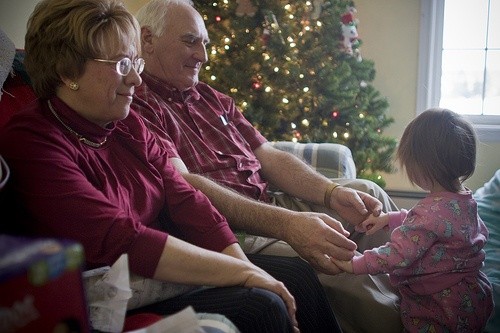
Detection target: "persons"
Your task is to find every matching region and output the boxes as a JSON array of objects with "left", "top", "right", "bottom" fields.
[
  {"left": 0, "top": 0, "right": 340, "bottom": 332},
  {"left": 130, "top": 0, "right": 403, "bottom": 332},
  {"left": 329, "top": 108, "right": 492, "bottom": 333}
]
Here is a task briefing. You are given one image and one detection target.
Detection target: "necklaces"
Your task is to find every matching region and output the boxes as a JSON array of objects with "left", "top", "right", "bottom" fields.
[{"left": 47, "top": 95, "right": 107, "bottom": 149}]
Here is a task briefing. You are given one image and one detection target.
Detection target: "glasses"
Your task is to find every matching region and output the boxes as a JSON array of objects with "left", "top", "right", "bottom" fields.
[{"left": 92, "top": 57, "right": 145, "bottom": 76}]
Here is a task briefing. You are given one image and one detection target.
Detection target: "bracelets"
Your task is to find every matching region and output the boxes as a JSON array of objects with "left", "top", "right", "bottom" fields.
[{"left": 324, "top": 182, "right": 341, "bottom": 208}]
